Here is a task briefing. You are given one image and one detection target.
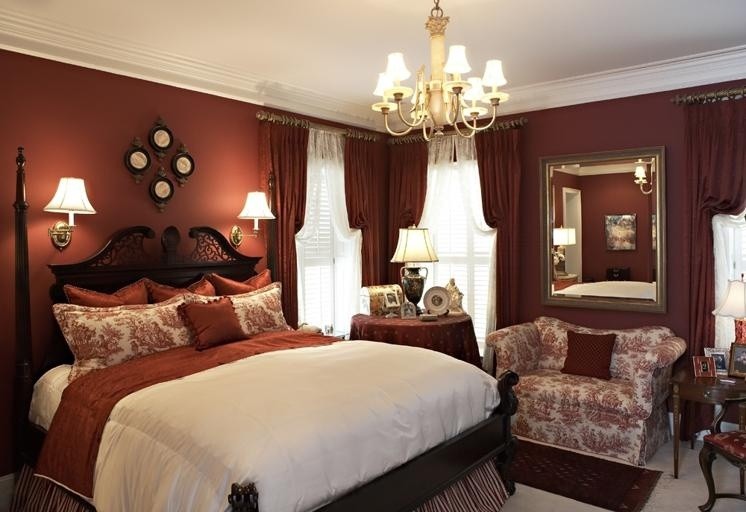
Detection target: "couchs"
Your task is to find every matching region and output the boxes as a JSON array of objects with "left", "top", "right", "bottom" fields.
[{"left": 485, "top": 316, "right": 687, "bottom": 468}]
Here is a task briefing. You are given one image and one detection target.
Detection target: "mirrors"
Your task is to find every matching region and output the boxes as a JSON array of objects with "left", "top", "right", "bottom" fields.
[{"left": 538, "top": 145, "right": 666, "bottom": 315}]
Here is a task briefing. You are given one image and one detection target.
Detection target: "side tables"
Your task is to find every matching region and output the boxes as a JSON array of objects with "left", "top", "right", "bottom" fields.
[{"left": 672, "top": 375, "right": 746, "bottom": 478}]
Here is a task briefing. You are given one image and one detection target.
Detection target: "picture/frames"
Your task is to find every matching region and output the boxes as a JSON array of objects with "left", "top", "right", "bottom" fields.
[
  {"left": 383, "top": 288, "right": 402, "bottom": 308},
  {"left": 694, "top": 357, "right": 716, "bottom": 377},
  {"left": 705, "top": 348, "right": 729, "bottom": 375},
  {"left": 652, "top": 213, "right": 656, "bottom": 250},
  {"left": 604, "top": 213, "right": 636, "bottom": 251},
  {"left": 730, "top": 342, "right": 746, "bottom": 377},
  {"left": 401, "top": 302, "right": 415, "bottom": 319}
]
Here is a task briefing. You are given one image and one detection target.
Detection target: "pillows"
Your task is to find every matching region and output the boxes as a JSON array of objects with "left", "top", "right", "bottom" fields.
[
  {"left": 148, "top": 274, "right": 219, "bottom": 303},
  {"left": 52, "top": 293, "right": 184, "bottom": 383},
  {"left": 213, "top": 268, "right": 272, "bottom": 297},
  {"left": 65, "top": 277, "right": 148, "bottom": 307},
  {"left": 560, "top": 331, "right": 617, "bottom": 381},
  {"left": 185, "top": 282, "right": 297, "bottom": 351},
  {"left": 554, "top": 276, "right": 579, "bottom": 291}
]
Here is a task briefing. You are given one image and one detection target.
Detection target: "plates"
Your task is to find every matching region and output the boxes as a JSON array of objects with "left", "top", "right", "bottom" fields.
[{"left": 424, "top": 287, "right": 451, "bottom": 315}]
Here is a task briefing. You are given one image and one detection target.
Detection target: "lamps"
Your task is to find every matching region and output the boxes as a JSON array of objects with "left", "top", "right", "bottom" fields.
[
  {"left": 711, "top": 274, "right": 745, "bottom": 344},
  {"left": 390, "top": 225, "right": 440, "bottom": 315},
  {"left": 370, "top": 0, "right": 510, "bottom": 142},
  {"left": 634, "top": 157, "right": 655, "bottom": 195},
  {"left": 44, "top": 176, "right": 97, "bottom": 251},
  {"left": 552, "top": 225, "right": 577, "bottom": 276},
  {"left": 229, "top": 191, "right": 277, "bottom": 247}
]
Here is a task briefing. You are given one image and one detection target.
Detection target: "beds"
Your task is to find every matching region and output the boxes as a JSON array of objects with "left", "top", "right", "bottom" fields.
[
  {"left": 16, "top": 144, "right": 517, "bottom": 512},
  {"left": 552, "top": 281, "right": 656, "bottom": 301}
]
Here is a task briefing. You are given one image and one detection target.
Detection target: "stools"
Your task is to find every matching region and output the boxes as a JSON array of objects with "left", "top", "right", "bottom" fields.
[{"left": 698, "top": 429, "right": 746, "bottom": 512}]
